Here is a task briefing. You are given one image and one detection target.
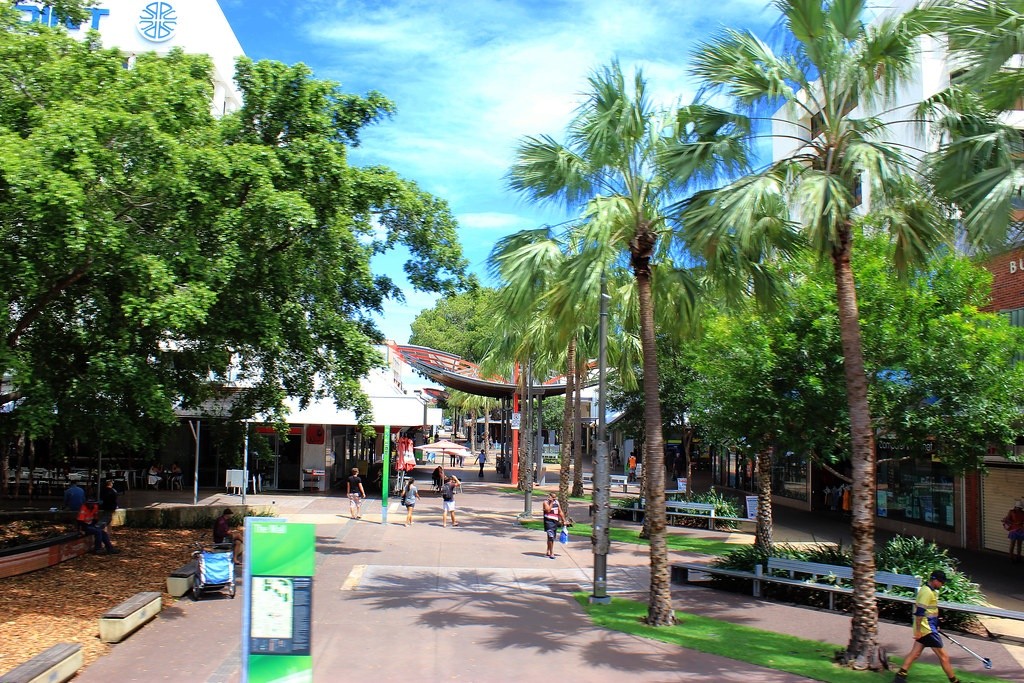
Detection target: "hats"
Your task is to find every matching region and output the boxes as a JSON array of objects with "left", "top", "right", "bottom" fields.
[
  {"left": 223, "top": 508, "right": 234, "bottom": 514},
  {"left": 86, "top": 498, "right": 98, "bottom": 504},
  {"left": 931, "top": 570, "right": 953, "bottom": 583}
]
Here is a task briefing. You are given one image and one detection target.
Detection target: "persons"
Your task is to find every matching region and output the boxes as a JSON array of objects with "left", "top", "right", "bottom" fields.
[
  {"left": 443, "top": 475, "right": 461, "bottom": 527},
  {"left": 401, "top": 477, "right": 420, "bottom": 527},
  {"left": 611, "top": 449, "right": 617, "bottom": 471},
  {"left": 435, "top": 432, "right": 439, "bottom": 442},
  {"left": 213, "top": 508, "right": 243, "bottom": 565},
  {"left": 422, "top": 434, "right": 428, "bottom": 444},
  {"left": 347, "top": 462, "right": 368, "bottom": 519},
  {"left": 673, "top": 453, "right": 682, "bottom": 476},
  {"left": 894, "top": 570, "right": 961, "bottom": 683},
  {"left": 1007, "top": 501, "right": 1024, "bottom": 560},
  {"left": 438, "top": 466, "right": 448, "bottom": 480},
  {"left": 450, "top": 454, "right": 463, "bottom": 468},
  {"left": 147, "top": 462, "right": 163, "bottom": 490},
  {"left": 629, "top": 454, "right": 636, "bottom": 482},
  {"left": 474, "top": 449, "right": 486, "bottom": 477},
  {"left": 171, "top": 461, "right": 181, "bottom": 489},
  {"left": 64, "top": 480, "right": 120, "bottom": 555},
  {"left": 543, "top": 493, "right": 566, "bottom": 559}
]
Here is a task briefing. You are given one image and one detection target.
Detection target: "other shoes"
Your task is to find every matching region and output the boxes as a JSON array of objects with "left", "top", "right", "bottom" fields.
[
  {"left": 549, "top": 555, "right": 555, "bottom": 559},
  {"left": 107, "top": 548, "right": 121, "bottom": 553},
  {"left": 442, "top": 525, "right": 446, "bottom": 527},
  {"left": 410, "top": 522, "right": 414, "bottom": 524},
  {"left": 351, "top": 516, "right": 362, "bottom": 519},
  {"left": 547, "top": 553, "right": 550, "bottom": 556},
  {"left": 452, "top": 523, "right": 459, "bottom": 526},
  {"left": 94, "top": 549, "right": 106, "bottom": 554},
  {"left": 233, "top": 561, "right": 241, "bottom": 565},
  {"left": 405, "top": 524, "right": 409, "bottom": 527}
]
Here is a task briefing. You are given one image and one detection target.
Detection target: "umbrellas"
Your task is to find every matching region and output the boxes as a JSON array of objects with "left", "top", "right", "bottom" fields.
[
  {"left": 413, "top": 439, "right": 467, "bottom": 486},
  {"left": 395, "top": 437, "right": 415, "bottom": 491}
]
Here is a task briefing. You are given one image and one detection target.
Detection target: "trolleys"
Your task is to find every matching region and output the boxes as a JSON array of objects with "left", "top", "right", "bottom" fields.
[{"left": 190, "top": 544, "right": 237, "bottom": 597}]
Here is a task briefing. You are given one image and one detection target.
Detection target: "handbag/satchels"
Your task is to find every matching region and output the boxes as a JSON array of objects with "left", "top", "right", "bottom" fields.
[
  {"left": 559, "top": 526, "right": 568, "bottom": 544},
  {"left": 401, "top": 495, "right": 406, "bottom": 506}
]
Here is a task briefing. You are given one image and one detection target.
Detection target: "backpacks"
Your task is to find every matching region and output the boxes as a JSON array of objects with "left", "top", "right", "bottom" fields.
[{"left": 441, "top": 484, "right": 453, "bottom": 499}]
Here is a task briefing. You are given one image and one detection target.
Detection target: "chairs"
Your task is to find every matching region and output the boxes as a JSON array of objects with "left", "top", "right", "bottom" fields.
[
  {"left": 145, "top": 471, "right": 184, "bottom": 492},
  {"left": 430, "top": 474, "right": 442, "bottom": 495}
]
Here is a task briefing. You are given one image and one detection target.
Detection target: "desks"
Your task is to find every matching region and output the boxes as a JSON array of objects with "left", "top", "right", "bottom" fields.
[{"left": 157, "top": 473, "right": 173, "bottom": 490}]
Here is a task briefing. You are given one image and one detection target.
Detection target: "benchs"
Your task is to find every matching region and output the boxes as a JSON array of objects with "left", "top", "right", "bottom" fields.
[
  {"left": 610, "top": 498, "right": 758, "bottom": 530},
  {"left": 0, "top": 642, "right": 83, "bottom": 683},
  {"left": 166, "top": 542, "right": 242, "bottom": 596},
  {"left": 98, "top": 591, "right": 163, "bottom": 643},
  {"left": 542, "top": 453, "right": 558, "bottom": 463},
  {"left": 669, "top": 557, "right": 1024, "bottom": 621},
  {"left": 582, "top": 473, "right": 641, "bottom": 493}
]
